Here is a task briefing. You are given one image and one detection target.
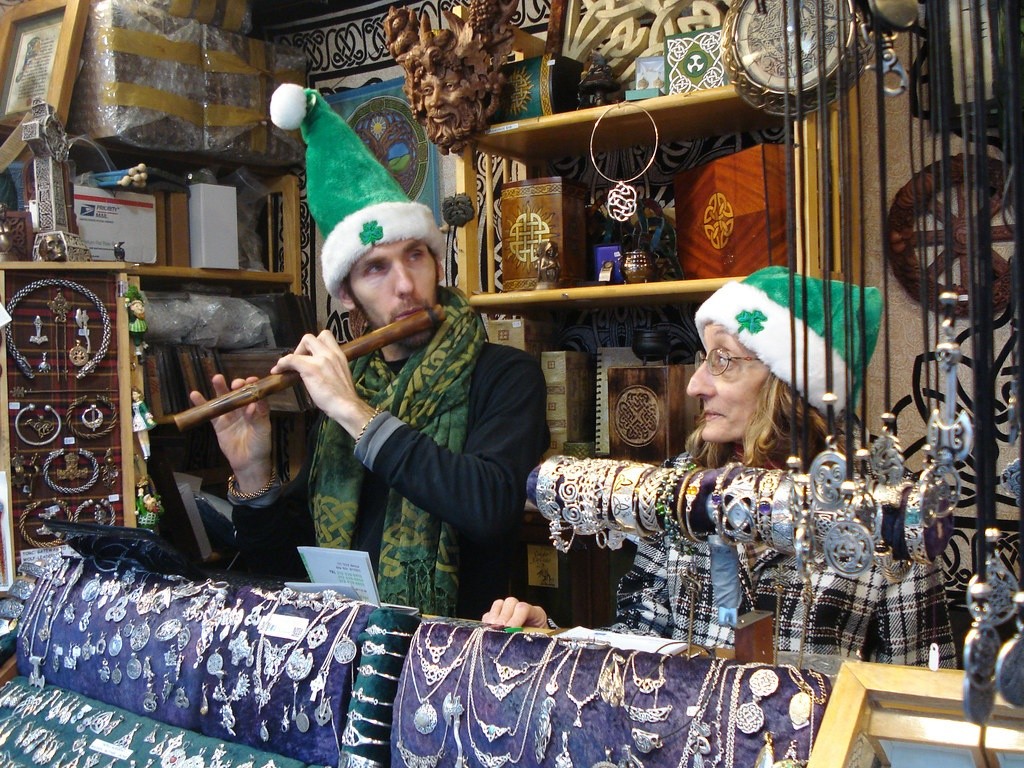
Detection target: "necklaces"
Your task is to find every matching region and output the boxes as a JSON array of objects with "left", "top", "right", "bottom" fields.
[
  {"left": 253, "top": 587, "right": 360, "bottom": 719},
  {"left": 16, "top": 551, "right": 86, "bottom": 689},
  {"left": 589, "top": 103, "right": 658, "bottom": 223},
  {"left": 778, "top": 0, "right": 1023, "bottom": 726},
  {"left": 397, "top": 621, "right": 827, "bottom": 768}
]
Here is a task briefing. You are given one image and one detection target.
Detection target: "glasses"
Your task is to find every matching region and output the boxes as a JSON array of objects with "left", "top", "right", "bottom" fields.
[{"left": 694, "top": 347, "right": 760, "bottom": 376}]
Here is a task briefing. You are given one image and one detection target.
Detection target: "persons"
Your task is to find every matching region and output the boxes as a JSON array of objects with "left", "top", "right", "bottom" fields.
[
  {"left": 385, "top": 1, "right": 517, "bottom": 156},
  {"left": 482, "top": 269, "right": 958, "bottom": 671},
  {"left": 190, "top": 239, "right": 549, "bottom": 618}
]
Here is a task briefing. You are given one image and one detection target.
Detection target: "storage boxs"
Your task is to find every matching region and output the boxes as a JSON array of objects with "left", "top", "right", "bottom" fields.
[
  {"left": 674, "top": 143, "right": 796, "bottom": 279},
  {"left": 502, "top": 176, "right": 588, "bottom": 292},
  {"left": 115, "top": 189, "right": 167, "bottom": 266},
  {"left": 189, "top": 184, "right": 240, "bottom": 269},
  {"left": 165, "top": 192, "right": 190, "bottom": 269},
  {"left": 29, "top": 184, "right": 157, "bottom": 264},
  {"left": 490, "top": 318, "right": 701, "bottom": 467},
  {"left": 67, "top": 0, "right": 307, "bottom": 161}
]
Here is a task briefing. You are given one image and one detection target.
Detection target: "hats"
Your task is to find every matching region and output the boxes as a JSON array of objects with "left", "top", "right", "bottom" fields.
[
  {"left": 695, "top": 266, "right": 884, "bottom": 420},
  {"left": 269, "top": 84, "right": 445, "bottom": 301}
]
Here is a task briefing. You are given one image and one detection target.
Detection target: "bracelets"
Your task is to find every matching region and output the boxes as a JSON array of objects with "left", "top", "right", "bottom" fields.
[
  {"left": 228, "top": 466, "right": 277, "bottom": 498},
  {"left": 337, "top": 624, "right": 414, "bottom": 768},
  {"left": 355, "top": 404, "right": 384, "bottom": 448},
  {"left": 535, "top": 453, "right": 942, "bottom": 565},
  {"left": 15, "top": 280, "right": 117, "bottom": 547}
]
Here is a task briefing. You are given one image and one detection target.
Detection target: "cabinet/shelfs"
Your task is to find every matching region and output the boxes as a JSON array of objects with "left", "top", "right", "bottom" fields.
[
  {"left": 459, "top": 84, "right": 864, "bottom": 513},
  {"left": 0, "top": 126, "right": 304, "bottom": 298}
]
